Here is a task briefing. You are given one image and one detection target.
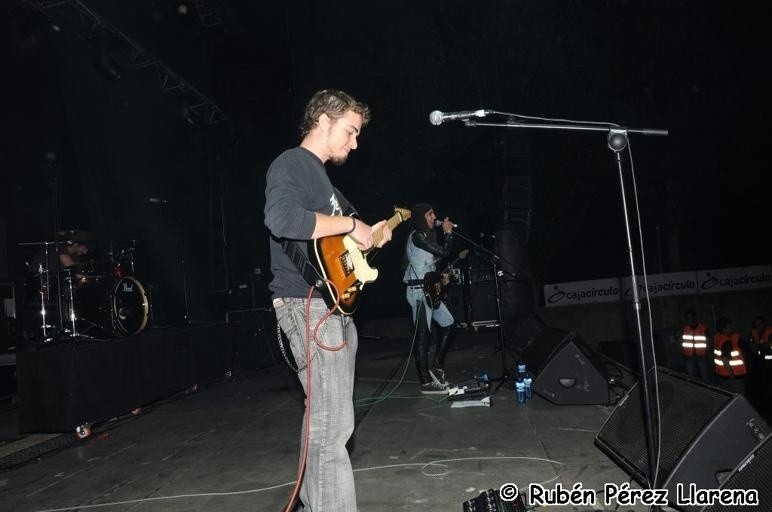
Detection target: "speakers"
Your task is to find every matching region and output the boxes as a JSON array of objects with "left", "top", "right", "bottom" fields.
[
  {"left": 700, "top": 432, "right": 772, "bottom": 511},
  {"left": 531, "top": 332, "right": 612, "bottom": 405},
  {"left": 519, "top": 326, "right": 600, "bottom": 375},
  {"left": 238, "top": 310, "right": 295, "bottom": 370},
  {"left": 593, "top": 364, "right": 772, "bottom": 512},
  {"left": 500, "top": 311, "right": 545, "bottom": 350}
]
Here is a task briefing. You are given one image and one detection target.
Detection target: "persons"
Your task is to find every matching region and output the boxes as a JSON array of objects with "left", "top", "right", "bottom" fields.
[
  {"left": 30, "top": 239, "right": 91, "bottom": 286},
  {"left": 403, "top": 203, "right": 458, "bottom": 394},
  {"left": 674, "top": 309, "right": 710, "bottom": 384},
  {"left": 751, "top": 315, "right": 772, "bottom": 363},
  {"left": 264, "top": 89, "right": 392, "bottom": 512},
  {"left": 711, "top": 317, "right": 751, "bottom": 396}
]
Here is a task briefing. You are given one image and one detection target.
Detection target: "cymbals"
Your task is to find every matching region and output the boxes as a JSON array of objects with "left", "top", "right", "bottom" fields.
[
  {"left": 114, "top": 237, "right": 153, "bottom": 245},
  {"left": 50, "top": 228, "right": 96, "bottom": 242}
]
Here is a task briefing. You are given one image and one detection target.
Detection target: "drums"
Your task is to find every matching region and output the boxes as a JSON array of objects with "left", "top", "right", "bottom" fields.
[
  {"left": 111, "top": 258, "right": 131, "bottom": 277},
  {"left": 98, "top": 275, "right": 148, "bottom": 338},
  {"left": 23, "top": 272, "right": 58, "bottom": 309},
  {"left": 62, "top": 275, "right": 80, "bottom": 289},
  {"left": 77, "top": 273, "right": 104, "bottom": 286}
]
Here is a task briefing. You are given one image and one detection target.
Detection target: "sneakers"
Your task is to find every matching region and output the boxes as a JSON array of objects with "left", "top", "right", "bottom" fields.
[{"left": 420, "top": 364, "right": 458, "bottom": 395}]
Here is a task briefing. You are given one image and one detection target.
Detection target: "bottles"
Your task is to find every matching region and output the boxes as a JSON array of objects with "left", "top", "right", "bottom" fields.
[{"left": 515, "top": 363, "right": 533, "bottom": 404}]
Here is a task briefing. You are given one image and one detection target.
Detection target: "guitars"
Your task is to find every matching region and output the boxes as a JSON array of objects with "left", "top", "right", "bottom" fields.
[
  {"left": 423, "top": 249, "right": 470, "bottom": 310},
  {"left": 308, "top": 208, "right": 413, "bottom": 317}
]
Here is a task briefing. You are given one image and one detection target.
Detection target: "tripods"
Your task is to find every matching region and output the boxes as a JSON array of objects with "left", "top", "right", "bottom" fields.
[{"left": 485, "top": 264, "right": 517, "bottom": 395}]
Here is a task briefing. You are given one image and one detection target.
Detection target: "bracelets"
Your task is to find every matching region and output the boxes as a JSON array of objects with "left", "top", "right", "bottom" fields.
[
  {"left": 346, "top": 215, "right": 357, "bottom": 234},
  {"left": 443, "top": 230, "right": 452, "bottom": 235}
]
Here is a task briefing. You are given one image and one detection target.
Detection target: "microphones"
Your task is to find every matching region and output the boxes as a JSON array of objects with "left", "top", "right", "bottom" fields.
[
  {"left": 429, "top": 108, "right": 494, "bottom": 126},
  {"left": 435, "top": 220, "right": 458, "bottom": 228}
]
